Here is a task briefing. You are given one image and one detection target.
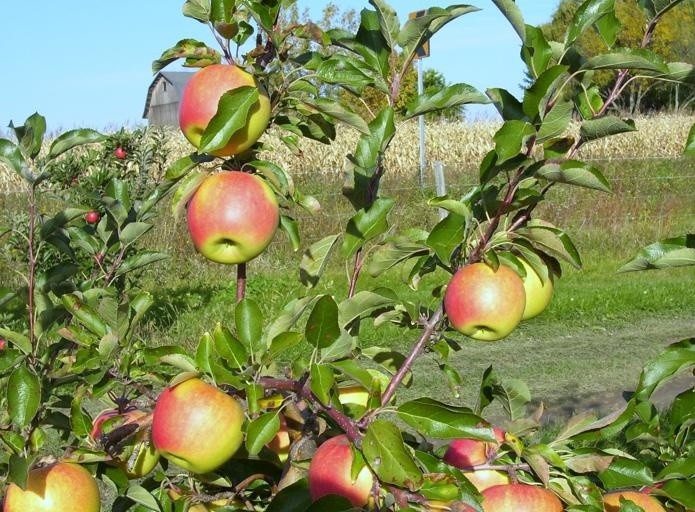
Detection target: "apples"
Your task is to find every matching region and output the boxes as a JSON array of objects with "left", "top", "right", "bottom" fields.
[
  {"left": 186, "top": 171, "right": 279, "bottom": 264},
  {"left": 151, "top": 378, "right": 243, "bottom": 473},
  {"left": 413, "top": 469, "right": 664, "bottom": 512},
  {"left": 438, "top": 423, "right": 506, "bottom": 466},
  {"left": 444, "top": 257, "right": 553, "bottom": 341},
  {"left": 180, "top": 66, "right": 271, "bottom": 156},
  {"left": 87, "top": 210, "right": 98, "bottom": 223},
  {"left": 3, "top": 462, "right": 102, "bottom": 512},
  {"left": 114, "top": 148, "right": 126, "bottom": 157},
  {"left": 311, "top": 431, "right": 373, "bottom": 510}
]
